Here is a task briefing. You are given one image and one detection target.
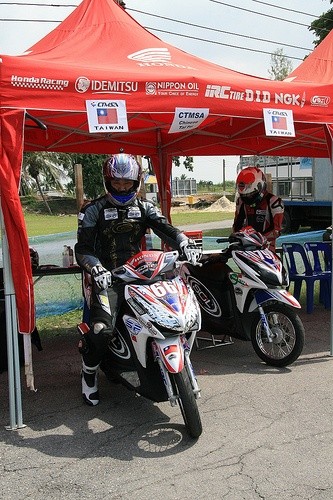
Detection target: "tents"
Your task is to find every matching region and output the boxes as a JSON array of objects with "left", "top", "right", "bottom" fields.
[{"left": 0, "top": 3, "right": 333, "bottom": 432}]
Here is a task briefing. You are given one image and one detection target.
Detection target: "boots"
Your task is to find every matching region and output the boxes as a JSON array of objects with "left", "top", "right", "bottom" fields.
[{"left": 79, "top": 358, "right": 102, "bottom": 406}]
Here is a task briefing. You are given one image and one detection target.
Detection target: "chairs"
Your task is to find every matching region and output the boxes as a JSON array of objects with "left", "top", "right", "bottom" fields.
[
  {"left": 282, "top": 243, "right": 329, "bottom": 313},
  {"left": 304, "top": 242, "right": 332, "bottom": 308}
]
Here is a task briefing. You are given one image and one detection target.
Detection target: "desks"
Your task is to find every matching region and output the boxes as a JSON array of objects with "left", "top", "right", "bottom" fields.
[{"left": 23, "top": 265, "right": 83, "bottom": 350}]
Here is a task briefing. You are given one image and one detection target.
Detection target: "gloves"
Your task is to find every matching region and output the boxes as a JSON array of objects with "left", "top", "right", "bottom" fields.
[
  {"left": 91, "top": 264, "right": 113, "bottom": 291},
  {"left": 179, "top": 238, "right": 204, "bottom": 267}
]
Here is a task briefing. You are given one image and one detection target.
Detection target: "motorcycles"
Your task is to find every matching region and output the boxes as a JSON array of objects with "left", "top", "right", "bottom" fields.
[
  {"left": 181, "top": 225, "right": 306, "bottom": 368},
  {"left": 77, "top": 248, "right": 210, "bottom": 439}
]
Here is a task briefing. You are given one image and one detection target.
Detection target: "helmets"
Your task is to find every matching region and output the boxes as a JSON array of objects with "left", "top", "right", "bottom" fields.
[
  {"left": 102, "top": 152, "right": 143, "bottom": 209},
  {"left": 236, "top": 167, "right": 268, "bottom": 206}
]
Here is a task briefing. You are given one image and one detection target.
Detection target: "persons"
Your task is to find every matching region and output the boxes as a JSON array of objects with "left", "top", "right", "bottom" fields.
[
  {"left": 72, "top": 153, "right": 203, "bottom": 408},
  {"left": 230, "top": 166, "right": 284, "bottom": 254}
]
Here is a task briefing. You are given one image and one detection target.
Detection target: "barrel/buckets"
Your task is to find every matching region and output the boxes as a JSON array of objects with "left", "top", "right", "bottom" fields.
[{"left": 188, "top": 196, "right": 193, "bottom": 204}]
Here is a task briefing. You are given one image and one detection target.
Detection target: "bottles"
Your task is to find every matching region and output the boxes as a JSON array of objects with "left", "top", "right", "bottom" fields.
[{"left": 61, "top": 244, "right": 73, "bottom": 267}]
